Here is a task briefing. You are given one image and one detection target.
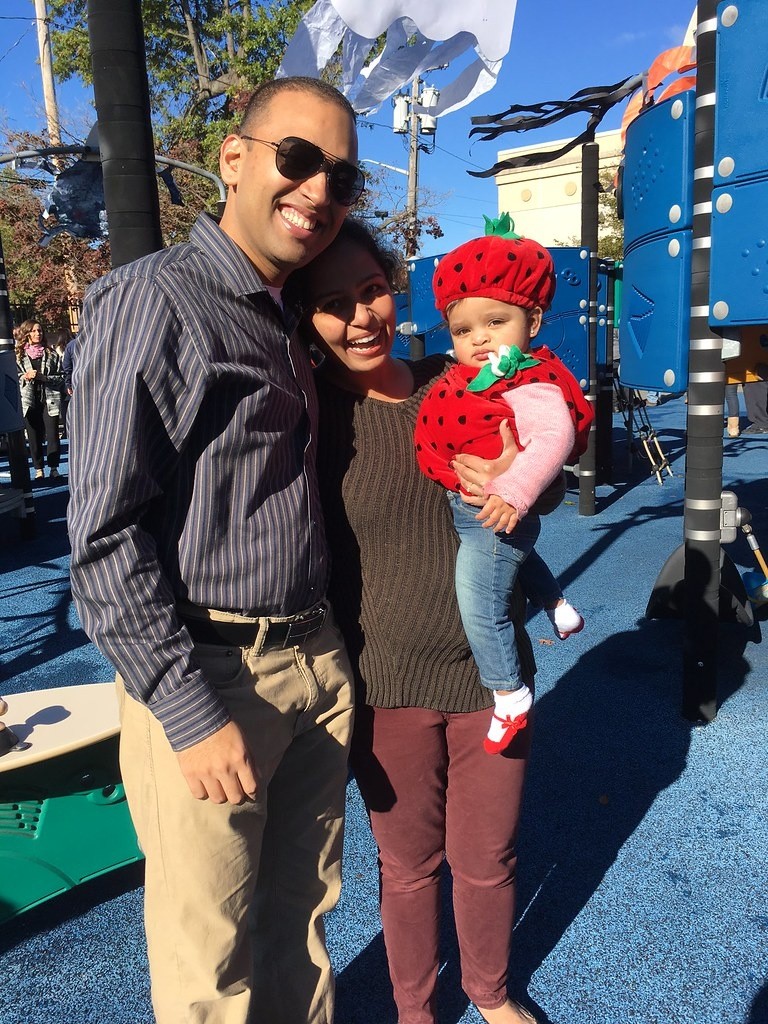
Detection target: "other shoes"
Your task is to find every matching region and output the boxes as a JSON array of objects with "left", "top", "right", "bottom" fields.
[
  {"left": 49, "top": 471, "right": 61, "bottom": 479},
  {"left": 554, "top": 608, "right": 584, "bottom": 641},
  {"left": 473, "top": 997, "right": 540, "bottom": 1024},
  {"left": 483, "top": 711, "right": 531, "bottom": 755},
  {"left": 35, "top": 469, "right": 45, "bottom": 479}
]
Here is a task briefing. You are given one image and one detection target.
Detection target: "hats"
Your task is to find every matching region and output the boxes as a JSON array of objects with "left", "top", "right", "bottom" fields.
[{"left": 430, "top": 211, "right": 555, "bottom": 311}]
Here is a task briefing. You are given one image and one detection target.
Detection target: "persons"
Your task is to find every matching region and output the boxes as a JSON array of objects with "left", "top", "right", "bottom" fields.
[
  {"left": 13, "top": 320, "right": 75, "bottom": 484},
  {"left": 412, "top": 237, "right": 580, "bottom": 753},
  {"left": 284, "top": 220, "right": 544, "bottom": 1024},
  {"left": 66, "top": 76, "right": 365, "bottom": 1024},
  {"left": 644, "top": 382, "right": 768, "bottom": 438}
]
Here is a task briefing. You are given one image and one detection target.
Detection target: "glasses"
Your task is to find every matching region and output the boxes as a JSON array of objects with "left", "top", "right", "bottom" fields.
[{"left": 238, "top": 135, "right": 367, "bottom": 207}]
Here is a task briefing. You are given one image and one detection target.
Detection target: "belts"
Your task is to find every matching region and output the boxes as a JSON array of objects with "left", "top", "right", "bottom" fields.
[{"left": 181, "top": 602, "right": 328, "bottom": 649}]
